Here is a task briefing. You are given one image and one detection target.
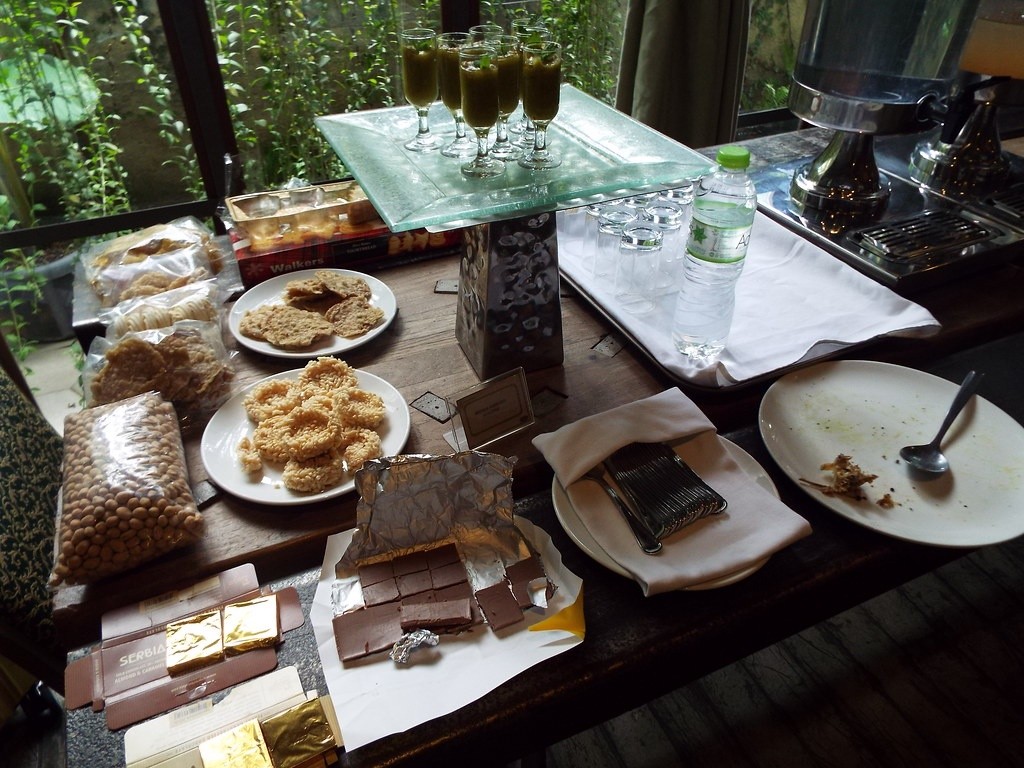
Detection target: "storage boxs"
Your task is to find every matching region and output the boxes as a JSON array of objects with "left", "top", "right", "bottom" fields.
[{"left": 230, "top": 215, "right": 466, "bottom": 289}]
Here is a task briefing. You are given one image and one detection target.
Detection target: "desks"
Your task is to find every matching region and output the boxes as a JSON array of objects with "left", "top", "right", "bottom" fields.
[{"left": 53, "top": 128, "right": 1024, "bottom": 768}]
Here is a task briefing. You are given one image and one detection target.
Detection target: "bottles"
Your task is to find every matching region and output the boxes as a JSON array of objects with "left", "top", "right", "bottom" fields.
[{"left": 670, "top": 146, "right": 757, "bottom": 361}]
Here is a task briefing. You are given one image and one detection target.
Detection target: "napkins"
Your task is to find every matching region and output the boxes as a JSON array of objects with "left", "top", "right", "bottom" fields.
[{"left": 529, "top": 386, "right": 813, "bottom": 598}]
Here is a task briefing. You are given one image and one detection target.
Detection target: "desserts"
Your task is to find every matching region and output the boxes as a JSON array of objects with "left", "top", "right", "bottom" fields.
[
  {"left": 236, "top": 356, "right": 387, "bottom": 492},
  {"left": 332, "top": 542, "right": 552, "bottom": 662}
]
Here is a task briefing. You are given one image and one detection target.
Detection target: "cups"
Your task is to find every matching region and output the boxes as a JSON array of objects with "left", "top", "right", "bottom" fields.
[{"left": 557, "top": 177, "right": 703, "bottom": 315}]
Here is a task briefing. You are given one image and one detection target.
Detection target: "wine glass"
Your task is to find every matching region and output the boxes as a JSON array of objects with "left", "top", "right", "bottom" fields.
[{"left": 400, "top": 20, "right": 564, "bottom": 180}]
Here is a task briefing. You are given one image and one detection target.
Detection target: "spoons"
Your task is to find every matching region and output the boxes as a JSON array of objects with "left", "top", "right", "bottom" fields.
[{"left": 899, "top": 370, "right": 985, "bottom": 475}]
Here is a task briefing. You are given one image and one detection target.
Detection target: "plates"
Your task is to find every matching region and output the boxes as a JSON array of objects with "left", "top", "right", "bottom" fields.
[
  {"left": 551, "top": 435, "right": 780, "bottom": 592},
  {"left": 760, "top": 359, "right": 1024, "bottom": 547},
  {"left": 200, "top": 369, "right": 411, "bottom": 506},
  {"left": 228, "top": 268, "right": 396, "bottom": 360}
]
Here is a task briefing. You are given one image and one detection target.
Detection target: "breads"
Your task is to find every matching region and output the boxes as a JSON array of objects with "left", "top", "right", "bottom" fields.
[{"left": 86, "top": 220, "right": 223, "bottom": 333}]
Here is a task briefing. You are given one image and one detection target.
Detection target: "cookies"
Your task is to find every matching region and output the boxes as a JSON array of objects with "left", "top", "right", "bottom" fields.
[
  {"left": 90, "top": 327, "right": 236, "bottom": 432},
  {"left": 240, "top": 271, "right": 385, "bottom": 349}
]
